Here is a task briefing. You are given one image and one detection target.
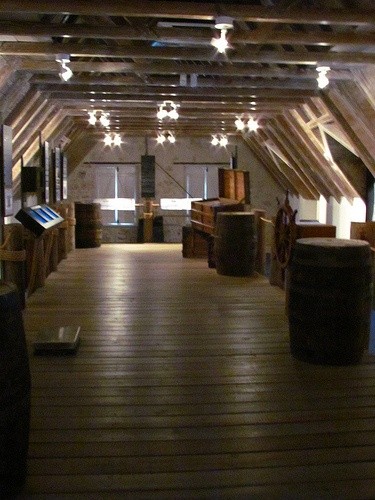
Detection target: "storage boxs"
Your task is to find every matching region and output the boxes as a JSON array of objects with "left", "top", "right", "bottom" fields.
[{"left": 191, "top": 167, "right": 250, "bottom": 236}]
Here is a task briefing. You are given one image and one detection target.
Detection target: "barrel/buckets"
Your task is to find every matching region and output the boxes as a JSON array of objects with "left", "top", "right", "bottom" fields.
[
  {"left": 0, "top": 282, "right": 31, "bottom": 496},
  {"left": 75, "top": 204, "right": 102, "bottom": 247},
  {"left": 287, "top": 238, "right": 372, "bottom": 362},
  {"left": 214, "top": 212, "right": 257, "bottom": 276}
]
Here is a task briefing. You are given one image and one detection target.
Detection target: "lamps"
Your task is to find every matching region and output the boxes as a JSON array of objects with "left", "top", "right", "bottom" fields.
[
  {"left": 210, "top": 16, "right": 234, "bottom": 54},
  {"left": 54, "top": 54, "right": 72, "bottom": 83}
]
